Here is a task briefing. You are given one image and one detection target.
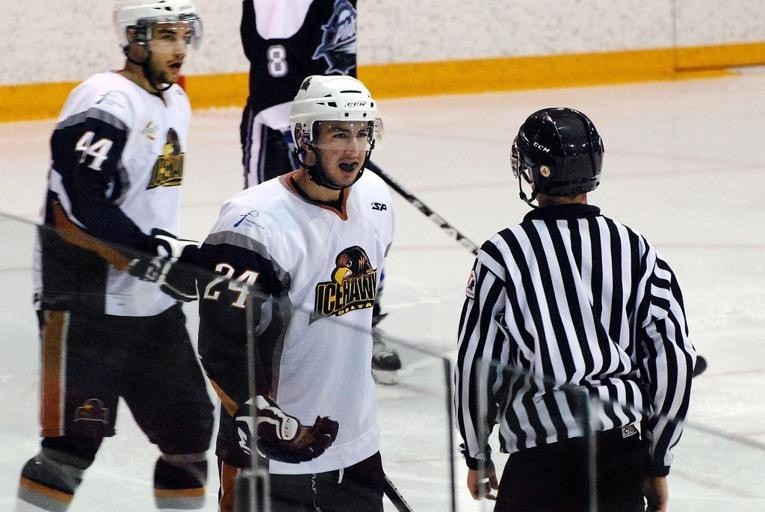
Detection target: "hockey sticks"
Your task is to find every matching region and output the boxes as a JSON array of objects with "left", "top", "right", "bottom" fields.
[{"left": 365, "top": 159, "right": 707, "bottom": 380}]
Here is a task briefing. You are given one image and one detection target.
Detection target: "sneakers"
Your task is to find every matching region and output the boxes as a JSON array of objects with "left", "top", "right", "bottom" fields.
[{"left": 370, "top": 327, "right": 401, "bottom": 371}]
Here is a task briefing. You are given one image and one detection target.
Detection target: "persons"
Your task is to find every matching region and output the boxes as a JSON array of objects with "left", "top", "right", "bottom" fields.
[
  {"left": 452, "top": 108, "right": 709, "bottom": 512},
  {"left": 16, "top": 2, "right": 214, "bottom": 512},
  {"left": 241, "top": 1, "right": 404, "bottom": 388},
  {"left": 196, "top": 73, "right": 390, "bottom": 511}
]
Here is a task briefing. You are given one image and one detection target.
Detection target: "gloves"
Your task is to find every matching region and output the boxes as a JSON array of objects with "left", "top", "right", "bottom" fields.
[
  {"left": 127, "top": 227, "right": 203, "bottom": 302},
  {"left": 234, "top": 391, "right": 338, "bottom": 461}
]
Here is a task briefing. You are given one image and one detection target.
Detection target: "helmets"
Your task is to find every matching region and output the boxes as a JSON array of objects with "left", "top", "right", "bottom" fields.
[
  {"left": 112, "top": 2, "right": 197, "bottom": 48},
  {"left": 289, "top": 76, "right": 383, "bottom": 149},
  {"left": 511, "top": 106, "right": 605, "bottom": 197}
]
